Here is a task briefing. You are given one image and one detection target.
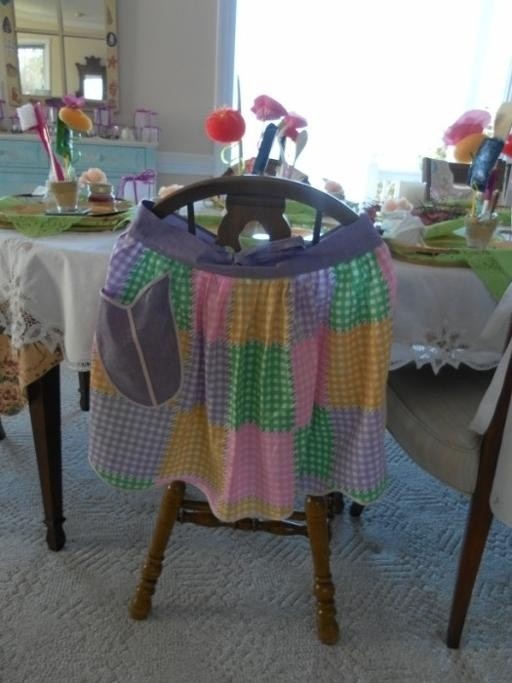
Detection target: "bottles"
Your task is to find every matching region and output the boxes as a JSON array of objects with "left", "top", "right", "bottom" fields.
[{"left": 88, "top": 183, "right": 114, "bottom": 213}]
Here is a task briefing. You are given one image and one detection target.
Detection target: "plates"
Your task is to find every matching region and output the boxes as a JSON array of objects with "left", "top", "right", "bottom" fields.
[{"left": 45, "top": 207, "right": 91, "bottom": 216}]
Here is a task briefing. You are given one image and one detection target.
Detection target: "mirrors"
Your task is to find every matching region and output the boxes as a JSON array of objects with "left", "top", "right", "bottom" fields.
[{"left": 1, "top": 0, "right": 121, "bottom": 111}]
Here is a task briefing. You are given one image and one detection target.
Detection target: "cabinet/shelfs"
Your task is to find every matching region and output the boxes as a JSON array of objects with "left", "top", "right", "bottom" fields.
[{"left": 0, "top": 138, "right": 159, "bottom": 195}]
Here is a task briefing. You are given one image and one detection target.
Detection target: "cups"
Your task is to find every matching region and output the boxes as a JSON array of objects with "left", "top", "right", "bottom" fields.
[
  {"left": 48, "top": 179, "right": 79, "bottom": 211},
  {"left": 399, "top": 180, "right": 426, "bottom": 207},
  {"left": 464, "top": 220, "right": 498, "bottom": 248}
]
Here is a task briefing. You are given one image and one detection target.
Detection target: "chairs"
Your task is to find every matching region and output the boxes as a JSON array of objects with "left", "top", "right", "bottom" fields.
[{"left": 102, "top": 174, "right": 402, "bottom": 647}]
